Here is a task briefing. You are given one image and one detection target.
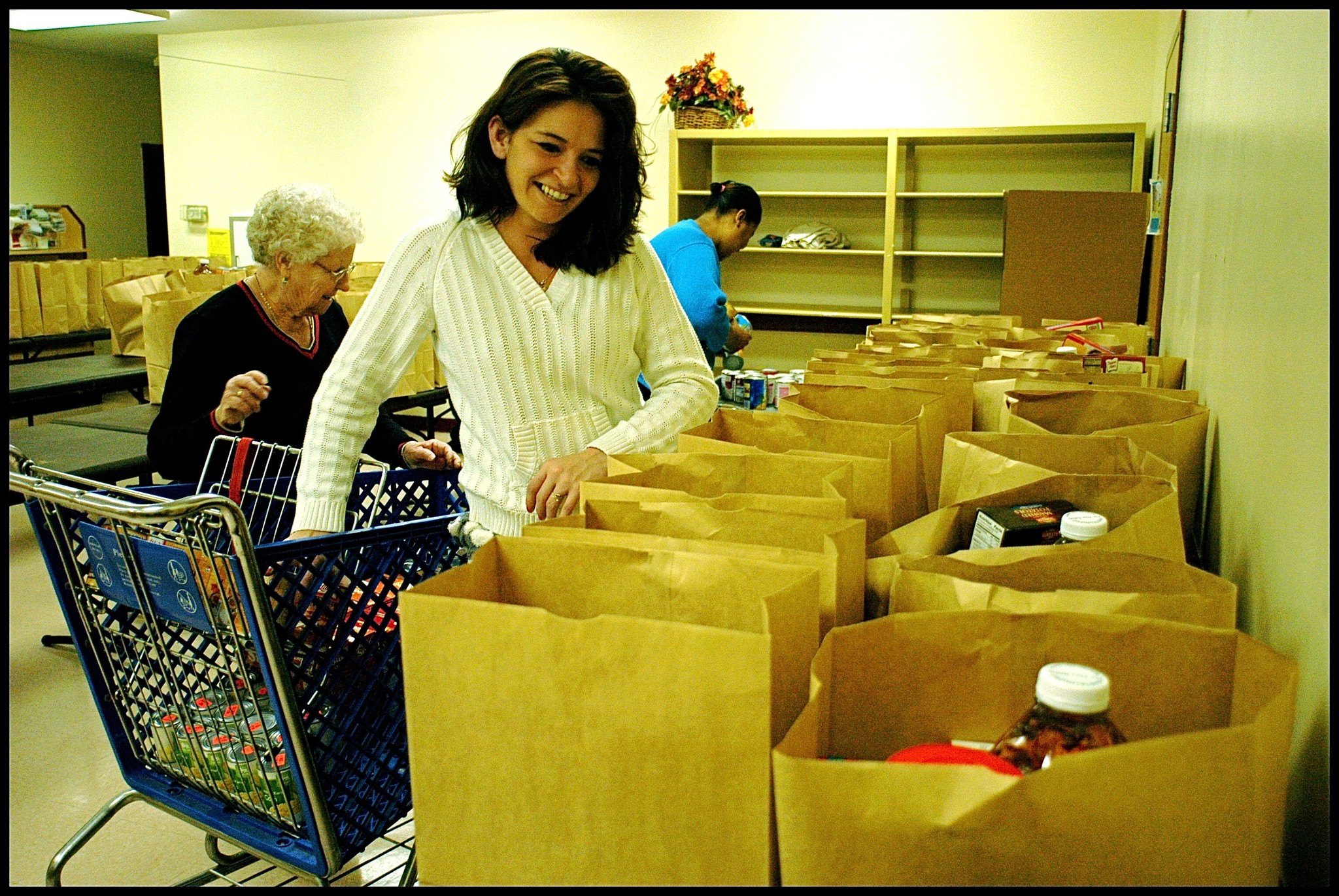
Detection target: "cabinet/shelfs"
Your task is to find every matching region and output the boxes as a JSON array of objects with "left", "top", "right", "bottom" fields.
[{"left": 669, "top": 122, "right": 1145, "bottom": 378}]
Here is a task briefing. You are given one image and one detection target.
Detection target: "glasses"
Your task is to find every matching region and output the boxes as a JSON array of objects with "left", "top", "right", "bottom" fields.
[{"left": 315, "top": 261, "right": 356, "bottom": 282}]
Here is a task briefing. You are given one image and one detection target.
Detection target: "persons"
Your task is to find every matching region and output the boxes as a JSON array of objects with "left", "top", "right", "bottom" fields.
[
  {"left": 636, "top": 182, "right": 762, "bottom": 401},
  {"left": 262, "top": 46, "right": 718, "bottom": 538},
  {"left": 150, "top": 184, "right": 463, "bottom": 545}
]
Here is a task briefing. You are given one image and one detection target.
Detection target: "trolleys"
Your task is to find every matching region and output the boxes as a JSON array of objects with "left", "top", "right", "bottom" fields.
[{"left": 9, "top": 429, "right": 470, "bottom": 888}]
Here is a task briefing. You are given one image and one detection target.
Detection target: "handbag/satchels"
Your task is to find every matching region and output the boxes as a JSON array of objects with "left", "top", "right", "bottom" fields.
[
  {"left": 396, "top": 313, "right": 1302, "bottom": 886},
  {"left": 8, "top": 251, "right": 256, "bottom": 405}
]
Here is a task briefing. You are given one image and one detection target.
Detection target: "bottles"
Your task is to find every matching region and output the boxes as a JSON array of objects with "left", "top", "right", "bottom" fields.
[
  {"left": 194, "top": 259, "right": 212, "bottom": 275},
  {"left": 1053, "top": 510, "right": 1109, "bottom": 545},
  {"left": 989, "top": 661, "right": 1128, "bottom": 773}
]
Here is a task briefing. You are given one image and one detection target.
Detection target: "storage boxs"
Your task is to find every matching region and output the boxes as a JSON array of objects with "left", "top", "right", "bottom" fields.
[{"left": 970, "top": 499, "right": 1078, "bottom": 549}]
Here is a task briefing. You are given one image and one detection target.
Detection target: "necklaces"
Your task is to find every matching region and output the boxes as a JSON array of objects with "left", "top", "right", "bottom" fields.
[
  {"left": 254, "top": 272, "right": 314, "bottom": 333},
  {"left": 537, "top": 267, "right": 555, "bottom": 291}
]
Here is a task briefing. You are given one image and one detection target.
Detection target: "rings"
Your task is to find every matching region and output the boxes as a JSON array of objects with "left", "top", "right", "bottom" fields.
[
  {"left": 552, "top": 493, "right": 563, "bottom": 501},
  {"left": 237, "top": 389, "right": 244, "bottom": 396}
]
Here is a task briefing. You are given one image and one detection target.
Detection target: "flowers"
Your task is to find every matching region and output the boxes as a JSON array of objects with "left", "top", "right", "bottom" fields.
[{"left": 648, "top": 51, "right": 756, "bottom": 134}]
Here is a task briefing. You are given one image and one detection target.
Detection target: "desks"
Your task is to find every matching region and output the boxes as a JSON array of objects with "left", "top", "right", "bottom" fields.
[{"left": 8, "top": 330, "right": 162, "bottom": 507}]
